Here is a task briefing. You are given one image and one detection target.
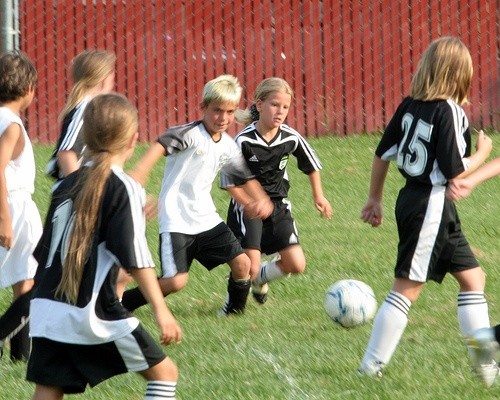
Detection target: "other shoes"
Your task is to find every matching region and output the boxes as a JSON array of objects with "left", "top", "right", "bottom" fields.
[
  {"left": 356, "top": 369, "right": 382, "bottom": 379},
  {"left": 476, "top": 360, "right": 497, "bottom": 388}
]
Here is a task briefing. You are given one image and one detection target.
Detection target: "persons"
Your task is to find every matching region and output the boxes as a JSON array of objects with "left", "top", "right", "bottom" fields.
[
  {"left": 23, "top": 94, "right": 183, "bottom": 400},
  {"left": 357, "top": 36, "right": 500, "bottom": 388},
  {"left": 0, "top": 50, "right": 133, "bottom": 366},
  {"left": 218, "top": 77, "right": 333, "bottom": 314},
  {"left": 122, "top": 74, "right": 274, "bottom": 317}
]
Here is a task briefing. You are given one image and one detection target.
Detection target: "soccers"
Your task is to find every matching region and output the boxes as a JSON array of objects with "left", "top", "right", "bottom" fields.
[{"left": 322, "top": 279, "right": 377, "bottom": 328}]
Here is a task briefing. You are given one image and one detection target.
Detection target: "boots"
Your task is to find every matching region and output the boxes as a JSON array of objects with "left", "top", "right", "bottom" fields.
[
  {"left": 10, "top": 320, "right": 30, "bottom": 362},
  {"left": 218, "top": 272, "right": 251, "bottom": 316},
  {"left": 120, "top": 278, "right": 167, "bottom": 314}
]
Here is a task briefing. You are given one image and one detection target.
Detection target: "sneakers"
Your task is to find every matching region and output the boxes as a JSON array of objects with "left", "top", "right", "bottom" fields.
[{"left": 252, "top": 282, "right": 268, "bottom": 304}]
